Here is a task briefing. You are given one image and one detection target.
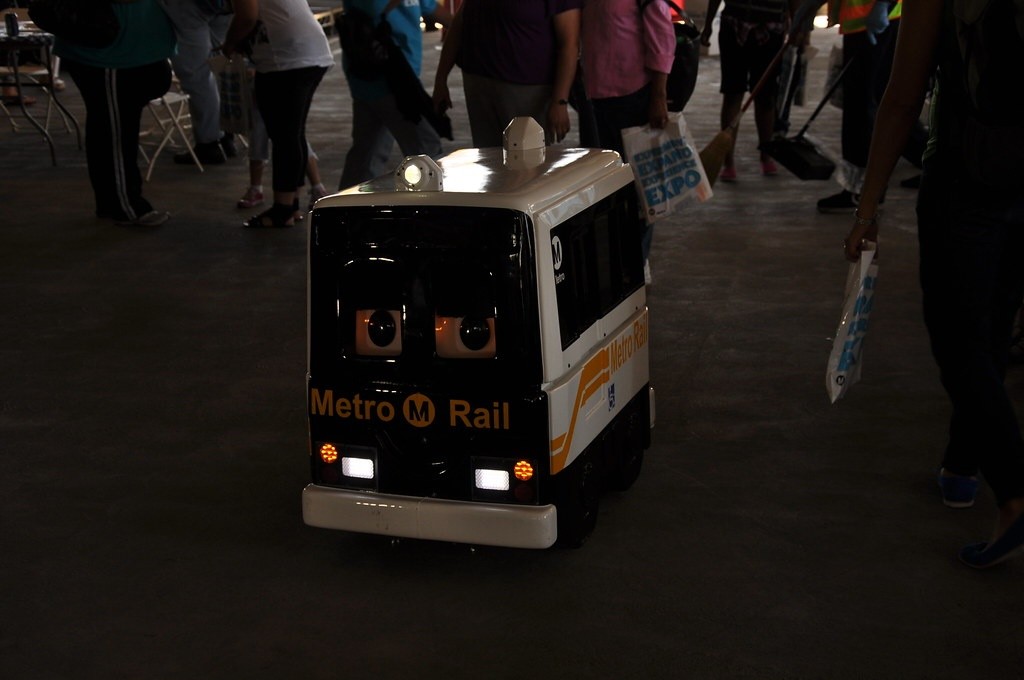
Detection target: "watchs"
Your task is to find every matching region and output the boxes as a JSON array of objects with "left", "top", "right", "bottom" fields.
[{"left": 552, "top": 97, "right": 569, "bottom": 105}]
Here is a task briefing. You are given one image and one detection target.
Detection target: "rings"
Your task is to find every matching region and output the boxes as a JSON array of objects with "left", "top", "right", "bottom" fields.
[{"left": 840, "top": 238, "right": 848, "bottom": 247}]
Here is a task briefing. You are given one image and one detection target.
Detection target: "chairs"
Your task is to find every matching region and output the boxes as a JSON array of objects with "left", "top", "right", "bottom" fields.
[{"left": 0, "top": 8, "right": 204, "bottom": 183}]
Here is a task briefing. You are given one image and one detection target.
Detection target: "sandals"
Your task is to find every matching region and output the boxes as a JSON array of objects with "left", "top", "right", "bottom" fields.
[{"left": 242, "top": 199, "right": 297, "bottom": 229}]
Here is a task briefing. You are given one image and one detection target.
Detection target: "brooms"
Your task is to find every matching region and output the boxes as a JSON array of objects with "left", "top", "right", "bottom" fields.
[{"left": 698, "top": 38, "right": 789, "bottom": 188}]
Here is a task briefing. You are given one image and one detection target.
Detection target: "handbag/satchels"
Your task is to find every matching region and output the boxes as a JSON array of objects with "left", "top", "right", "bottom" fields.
[
  {"left": 621, "top": 110, "right": 712, "bottom": 226},
  {"left": 642, "top": 1, "right": 702, "bottom": 112},
  {"left": 825, "top": 240, "right": 880, "bottom": 404}
]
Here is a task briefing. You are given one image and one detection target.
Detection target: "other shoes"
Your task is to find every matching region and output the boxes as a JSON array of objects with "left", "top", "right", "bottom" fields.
[
  {"left": 960, "top": 513, "right": 1024, "bottom": 568},
  {"left": 3, "top": 93, "right": 38, "bottom": 104},
  {"left": 221, "top": 135, "right": 235, "bottom": 156},
  {"left": 176, "top": 142, "right": 226, "bottom": 164},
  {"left": 41, "top": 77, "right": 65, "bottom": 90},
  {"left": 941, "top": 468, "right": 980, "bottom": 507}
]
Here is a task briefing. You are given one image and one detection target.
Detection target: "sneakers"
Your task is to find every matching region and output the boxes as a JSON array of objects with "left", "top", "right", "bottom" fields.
[
  {"left": 239, "top": 187, "right": 263, "bottom": 207},
  {"left": 720, "top": 166, "right": 735, "bottom": 180},
  {"left": 309, "top": 192, "right": 330, "bottom": 210},
  {"left": 901, "top": 174, "right": 921, "bottom": 189},
  {"left": 818, "top": 189, "right": 884, "bottom": 213},
  {"left": 115, "top": 209, "right": 169, "bottom": 226},
  {"left": 763, "top": 159, "right": 777, "bottom": 174}
]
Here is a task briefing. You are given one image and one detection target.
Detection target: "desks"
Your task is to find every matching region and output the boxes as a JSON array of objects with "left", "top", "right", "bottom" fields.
[{"left": 0, "top": 20, "right": 73, "bottom": 143}]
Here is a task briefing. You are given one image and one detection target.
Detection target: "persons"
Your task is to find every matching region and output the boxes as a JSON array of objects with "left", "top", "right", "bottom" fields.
[
  {"left": 845, "top": 0, "right": 1024, "bottom": 569},
  {"left": 28, "top": 0, "right": 178, "bottom": 228},
  {"left": 151, "top": 1, "right": 943, "bottom": 234}
]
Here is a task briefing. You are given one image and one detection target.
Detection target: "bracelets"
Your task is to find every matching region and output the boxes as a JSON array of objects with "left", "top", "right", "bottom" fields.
[{"left": 853, "top": 207, "right": 880, "bottom": 225}]
